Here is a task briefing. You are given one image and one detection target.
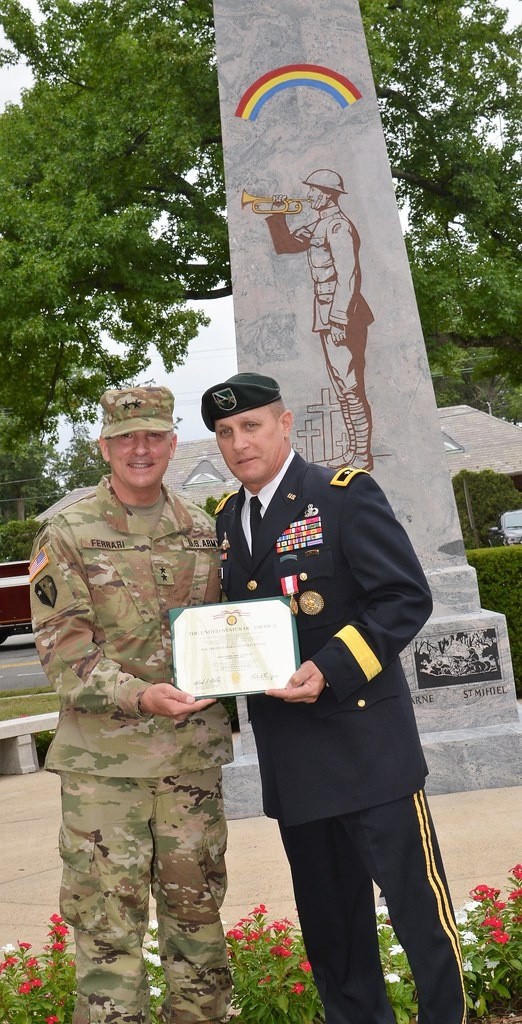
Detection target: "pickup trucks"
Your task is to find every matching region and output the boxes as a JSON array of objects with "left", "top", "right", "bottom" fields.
[{"left": 0, "top": 560, "right": 34, "bottom": 644}]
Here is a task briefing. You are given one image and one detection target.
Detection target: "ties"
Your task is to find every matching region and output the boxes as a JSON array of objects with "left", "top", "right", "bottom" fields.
[{"left": 250, "top": 496, "right": 263, "bottom": 557}]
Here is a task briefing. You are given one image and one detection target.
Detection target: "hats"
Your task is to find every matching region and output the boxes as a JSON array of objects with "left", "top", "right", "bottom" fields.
[
  {"left": 100, "top": 386, "right": 175, "bottom": 439},
  {"left": 201, "top": 372, "right": 281, "bottom": 432}
]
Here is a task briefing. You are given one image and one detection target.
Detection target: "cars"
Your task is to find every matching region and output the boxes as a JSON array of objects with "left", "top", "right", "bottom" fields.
[{"left": 486, "top": 510, "right": 522, "bottom": 547}]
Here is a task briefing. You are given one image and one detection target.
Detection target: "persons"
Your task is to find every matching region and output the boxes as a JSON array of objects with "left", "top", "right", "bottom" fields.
[
  {"left": 29, "top": 388, "right": 237, "bottom": 1022},
  {"left": 201, "top": 373, "right": 470, "bottom": 1024}
]
